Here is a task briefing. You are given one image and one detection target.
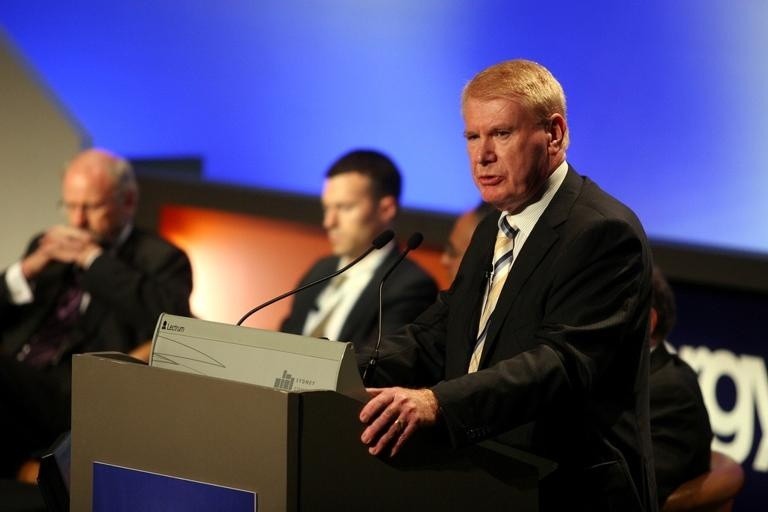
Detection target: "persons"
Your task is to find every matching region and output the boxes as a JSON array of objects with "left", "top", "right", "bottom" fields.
[
  {"left": 0, "top": 147, "right": 193, "bottom": 488},
  {"left": 437, "top": 197, "right": 497, "bottom": 286},
  {"left": 650, "top": 264, "right": 717, "bottom": 511},
  {"left": 353, "top": 58, "right": 660, "bottom": 511},
  {"left": 273, "top": 149, "right": 443, "bottom": 353}
]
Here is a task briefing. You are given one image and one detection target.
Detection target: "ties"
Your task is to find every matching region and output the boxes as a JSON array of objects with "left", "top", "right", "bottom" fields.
[
  {"left": 24, "top": 270, "right": 85, "bottom": 367},
  {"left": 468, "top": 214, "right": 518, "bottom": 375}
]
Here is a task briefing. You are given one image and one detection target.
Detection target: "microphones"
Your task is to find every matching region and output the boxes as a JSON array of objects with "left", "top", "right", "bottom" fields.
[
  {"left": 235, "top": 229, "right": 396, "bottom": 326},
  {"left": 361, "top": 231, "right": 425, "bottom": 384}
]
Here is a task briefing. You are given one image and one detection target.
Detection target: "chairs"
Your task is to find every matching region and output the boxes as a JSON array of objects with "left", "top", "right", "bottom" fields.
[{"left": 664, "top": 450, "right": 744, "bottom": 510}]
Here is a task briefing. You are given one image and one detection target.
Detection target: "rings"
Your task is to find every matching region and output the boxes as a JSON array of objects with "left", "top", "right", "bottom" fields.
[{"left": 394, "top": 416, "right": 405, "bottom": 428}]
